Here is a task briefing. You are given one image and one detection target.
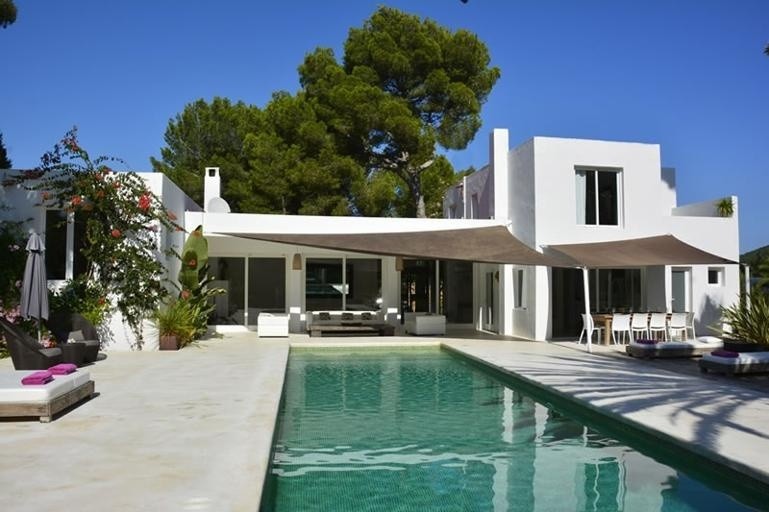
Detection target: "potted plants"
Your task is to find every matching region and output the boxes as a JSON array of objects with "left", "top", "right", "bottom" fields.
[{"left": 138, "top": 296, "right": 207, "bottom": 350}]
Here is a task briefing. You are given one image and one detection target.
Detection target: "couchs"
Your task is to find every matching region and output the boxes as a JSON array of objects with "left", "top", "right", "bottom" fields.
[{"left": 306, "top": 310, "right": 385, "bottom": 333}]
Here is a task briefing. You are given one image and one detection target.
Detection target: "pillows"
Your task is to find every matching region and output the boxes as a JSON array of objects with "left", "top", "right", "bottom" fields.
[
  {"left": 361, "top": 313, "right": 372, "bottom": 320},
  {"left": 319, "top": 313, "right": 330, "bottom": 320},
  {"left": 342, "top": 313, "right": 353, "bottom": 320},
  {"left": 698, "top": 336, "right": 723, "bottom": 343}
]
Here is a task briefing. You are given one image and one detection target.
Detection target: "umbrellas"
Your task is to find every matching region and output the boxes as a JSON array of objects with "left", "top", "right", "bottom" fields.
[{"left": 19, "top": 234, "right": 49, "bottom": 343}]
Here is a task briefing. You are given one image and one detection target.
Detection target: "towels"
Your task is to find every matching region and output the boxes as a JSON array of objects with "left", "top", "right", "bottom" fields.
[
  {"left": 48, "top": 364, "right": 76, "bottom": 374},
  {"left": 711, "top": 350, "right": 739, "bottom": 357},
  {"left": 22, "top": 372, "right": 53, "bottom": 385}
]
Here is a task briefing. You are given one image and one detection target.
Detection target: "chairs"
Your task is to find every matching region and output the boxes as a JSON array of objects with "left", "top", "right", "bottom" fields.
[
  {"left": 257, "top": 312, "right": 290, "bottom": 337},
  {"left": 578, "top": 307, "right": 696, "bottom": 347},
  {"left": 0, "top": 309, "right": 100, "bottom": 369},
  {"left": 699, "top": 352, "right": 769, "bottom": 377},
  {"left": 404, "top": 311, "right": 446, "bottom": 336},
  {"left": 626, "top": 340, "right": 725, "bottom": 361},
  {"left": 0, "top": 369, "right": 94, "bottom": 423}
]
